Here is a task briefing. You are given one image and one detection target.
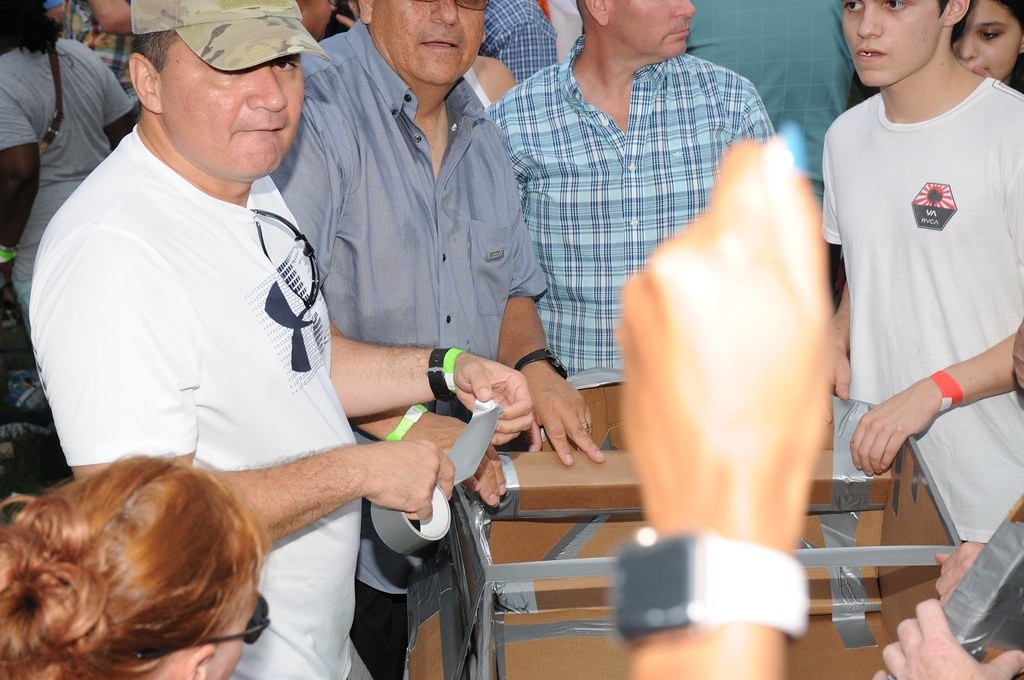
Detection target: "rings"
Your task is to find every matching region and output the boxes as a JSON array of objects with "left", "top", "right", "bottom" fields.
[{"left": 581, "top": 422, "right": 589, "bottom": 430}]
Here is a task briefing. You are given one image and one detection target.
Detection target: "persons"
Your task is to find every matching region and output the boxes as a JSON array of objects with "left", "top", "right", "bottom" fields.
[{"left": 0, "top": 0, "right": 1024, "bottom": 680}]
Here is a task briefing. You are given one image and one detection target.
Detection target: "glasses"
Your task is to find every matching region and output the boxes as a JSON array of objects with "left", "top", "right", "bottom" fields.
[
  {"left": 136, "top": 592, "right": 270, "bottom": 661},
  {"left": 412, "top": 0, "right": 490, "bottom": 11}
]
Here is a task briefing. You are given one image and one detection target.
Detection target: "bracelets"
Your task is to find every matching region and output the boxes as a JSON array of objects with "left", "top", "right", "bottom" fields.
[
  {"left": 930, "top": 371, "right": 963, "bottom": 412},
  {"left": 425, "top": 348, "right": 459, "bottom": 403},
  {"left": 443, "top": 345, "right": 465, "bottom": 394}
]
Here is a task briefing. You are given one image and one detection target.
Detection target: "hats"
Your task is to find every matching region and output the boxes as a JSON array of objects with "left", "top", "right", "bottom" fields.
[{"left": 128, "top": 0, "right": 332, "bottom": 73}]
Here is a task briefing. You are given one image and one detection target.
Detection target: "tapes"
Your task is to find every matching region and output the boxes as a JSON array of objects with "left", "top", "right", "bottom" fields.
[{"left": 370, "top": 484, "right": 452, "bottom": 557}]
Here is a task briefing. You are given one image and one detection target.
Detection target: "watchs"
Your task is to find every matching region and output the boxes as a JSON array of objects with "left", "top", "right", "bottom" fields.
[
  {"left": 612, "top": 532, "right": 811, "bottom": 640},
  {"left": 514, "top": 348, "right": 568, "bottom": 380}
]
